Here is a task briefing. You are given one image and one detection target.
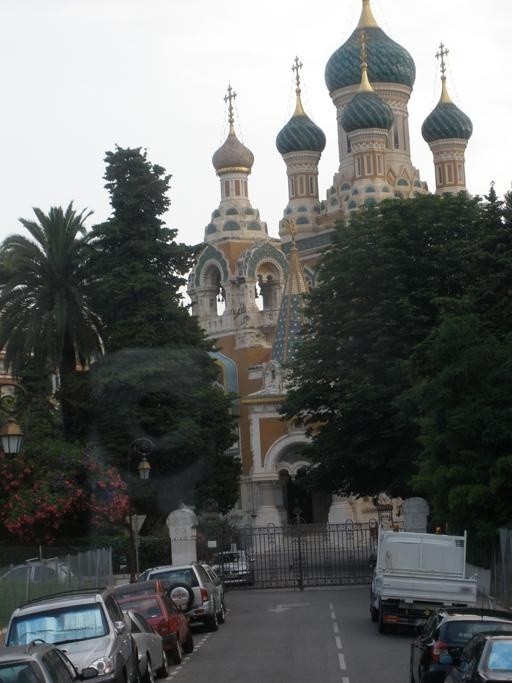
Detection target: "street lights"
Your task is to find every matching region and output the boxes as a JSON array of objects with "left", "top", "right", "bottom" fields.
[
  {"left": 127, "top": 515, "right": 148, "bottom": 583},
  {"left": 127, "top": 436, "right": 155, "bottom": 583}
]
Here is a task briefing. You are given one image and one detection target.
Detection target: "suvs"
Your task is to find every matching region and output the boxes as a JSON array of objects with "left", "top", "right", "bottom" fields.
[
  {"left": 4, "top": 586, "right": 139, "bottom": 683},
  {"left": 146, "top": 565, "right": 225, "bottom": 630}
]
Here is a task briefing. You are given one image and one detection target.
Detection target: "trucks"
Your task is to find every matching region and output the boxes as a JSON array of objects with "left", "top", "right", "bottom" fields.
[{"left": 369, "top": 524, "right": 477, "bottom": 635}]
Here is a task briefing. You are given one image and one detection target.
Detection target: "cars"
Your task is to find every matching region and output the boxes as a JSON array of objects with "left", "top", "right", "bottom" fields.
[
  {"left": 114, "top": 579, "right": 163, "bottom": 600},
  {"left": 410, "top": 606, "right": 512, "bottom": 683},
  {"left": 120, "top": 609, "right": 170, "bottom": 683},
  {"left": 0, "top": 638, "right": 98, "bottom": 683},
  {"left": 211, "top": 550, "right": 255, "bottom": 586},
  {"left": 0, "top": 556, "right": 74, "bottom": 582},
  {"left": 444, "top": 630, "right": 512, "bottom": 683},
  {"left": 116, "top": 593, "right": 194, "bottom": 664}
]
